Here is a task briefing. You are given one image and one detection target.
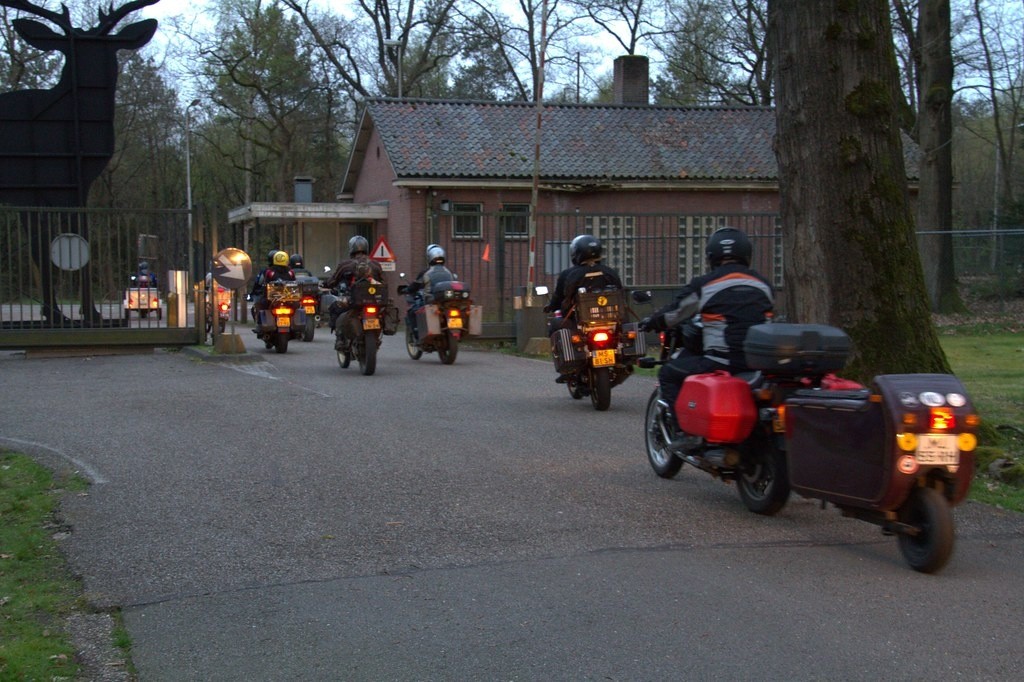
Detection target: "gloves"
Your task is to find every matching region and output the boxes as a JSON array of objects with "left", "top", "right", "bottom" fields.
[
  {"left": 542, "top": 305, "right": 550, "bottom": 314},
  {"left": 638, "top": 316, "right": 655, "bottom": 333},
  {"left": 401, "top": 288, "right": 409, "bottom": 295}
]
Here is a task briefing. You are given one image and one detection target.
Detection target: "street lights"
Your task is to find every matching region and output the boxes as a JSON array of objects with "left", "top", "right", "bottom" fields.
[
  {"left": 383, "top": 39, "right": 402, "bottom": 98},
  {"left": 185, "top": 99, "right": 201, "bottom": 301}
]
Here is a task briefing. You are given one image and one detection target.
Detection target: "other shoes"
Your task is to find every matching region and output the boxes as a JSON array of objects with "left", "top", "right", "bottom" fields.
[
  {"left": 335, "top": 335, "right": 343, "bottom": 350},
  {"left": 251, "top": 324, "right": 262, "bottom": 333},
  {"left": 556, "top": 370, "right": 575, "bottom": 382}
]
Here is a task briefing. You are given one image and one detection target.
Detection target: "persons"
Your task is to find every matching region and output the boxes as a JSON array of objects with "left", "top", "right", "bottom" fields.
[
  {"left": 404, "top": 244, "right": 454, "bottom": 347},
  {"left": 542, "top": 235, "right": 623, "bottom": 385},
  {"left": 205, "top": 260, "right": 213, "bottom": 289},
  {"left": 324, "top": 236, "right": 382, "bottom": 349},
  {"left": 636, "top": 226, "right": 776, "bottom": 449},
  {"left": 247, "top": 250, "right": 311, "bottom": 333},
  {"left": 138, "top": 262, "right": 155, "bottom": 289}
]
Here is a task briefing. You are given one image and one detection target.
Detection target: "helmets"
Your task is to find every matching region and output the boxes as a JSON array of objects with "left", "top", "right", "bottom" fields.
[
  {"left": 268, "top": 249, "right": 279, "bottom": 264},
  {"left": 425, "top": 244, "right": 446, "bottom": 265},
  {"left": 290, "top": 254, "right": 303, "bottom": 268},
  {"left": 574, "top": 236, "right": 604, "bottom": 266},
  {"left": 273, "top": 251, "right": 289, "bottom": 267},
  {"left": 139, "top": 262, "right": 148, "bottom": 269},
  {"left": 569, "top": 235, "right": 590, "bottom": 264},
  {"left": 349, "top": 235, "right": 369, "bottom": 257},
  {"left": 704, "top": 226, "right": 753, "bottom": 270}
]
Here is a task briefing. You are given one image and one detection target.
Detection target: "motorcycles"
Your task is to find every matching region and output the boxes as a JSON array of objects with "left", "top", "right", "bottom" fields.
[
  {"left": 241, "top": 275, "right": 319, "bottom": 353},
  {"left": 535, "top": 286, "right": 647, "bottom": 412},
  {"left": 399, "top": 273, "right": 483, "bottom": 365},
  {"left": 205, "top": 286, "right": 235, "bottom": 334},
  {"left": 623, "top": 287, "right": 850, "bottom": 520},
  {"left": 320, "top": 264, "right": 399, "bottom": 375},
  {"left": 124, "top": 274, "right": 163, "bottom": 321}
]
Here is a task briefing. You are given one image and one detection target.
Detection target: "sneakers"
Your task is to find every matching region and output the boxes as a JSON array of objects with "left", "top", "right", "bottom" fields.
[{"left": 669, "top": 434, "right": 702, "bottom": 450}]
